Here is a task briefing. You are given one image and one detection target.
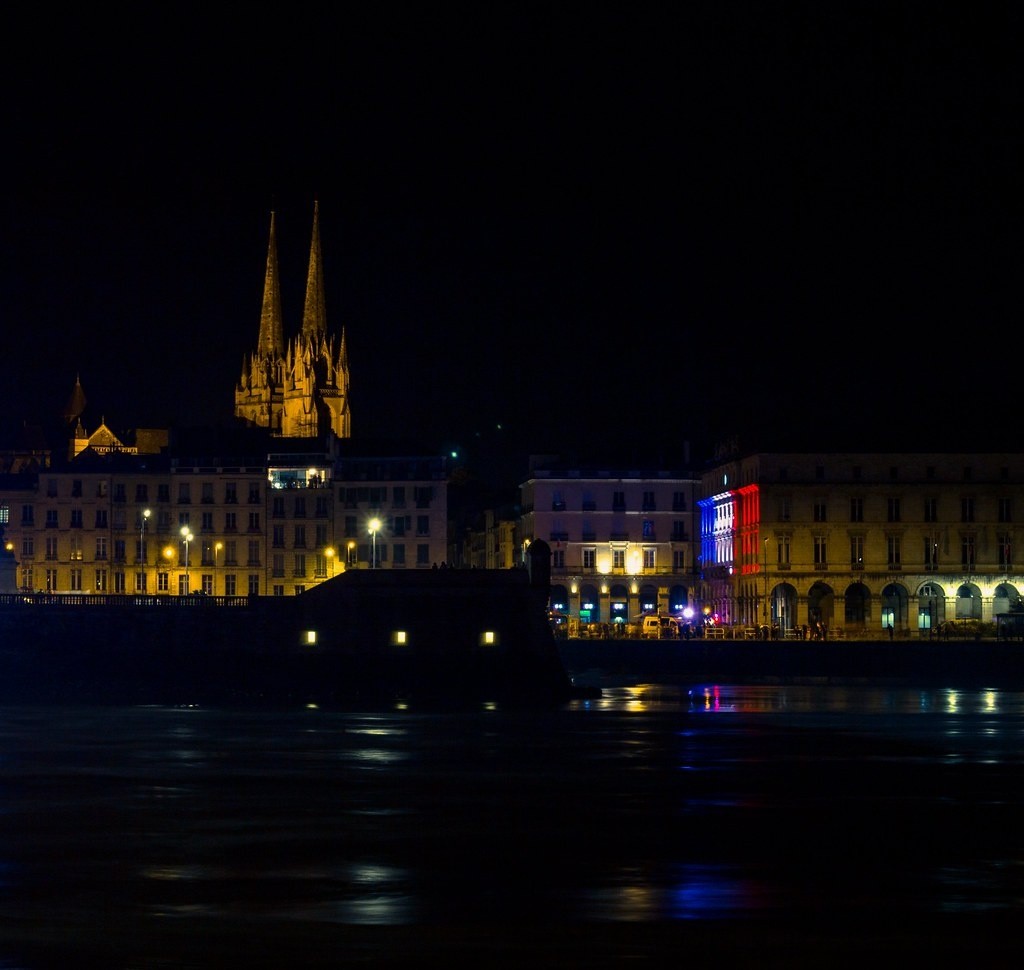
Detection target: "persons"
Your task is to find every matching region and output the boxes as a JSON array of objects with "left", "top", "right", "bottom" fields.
[
  {"left": 936, "top": 625, "right": 941, "bottom": 641},
  {"left": 944, "top": 625, "right": 949, "bottom": 641},
  {"left": 546, "top": 616, "right": 827, "bottom": 643},
  {"left": 887, "top": 624, "right": 894, "bottom": 642}
]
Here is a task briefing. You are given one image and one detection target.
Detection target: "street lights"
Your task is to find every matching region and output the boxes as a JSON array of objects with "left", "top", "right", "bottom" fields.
[
  {"left": 364, "top": 514, "right": 386, "bottom": 569},
  {"left": 345, "top": 541, "right": 357, "bottom": 570},
  {"left": 139, "top": 508, "right": 152, "bottom": 595},
  {"left": 177, "top": 524, "right": 197, "bottom": 599}
]
